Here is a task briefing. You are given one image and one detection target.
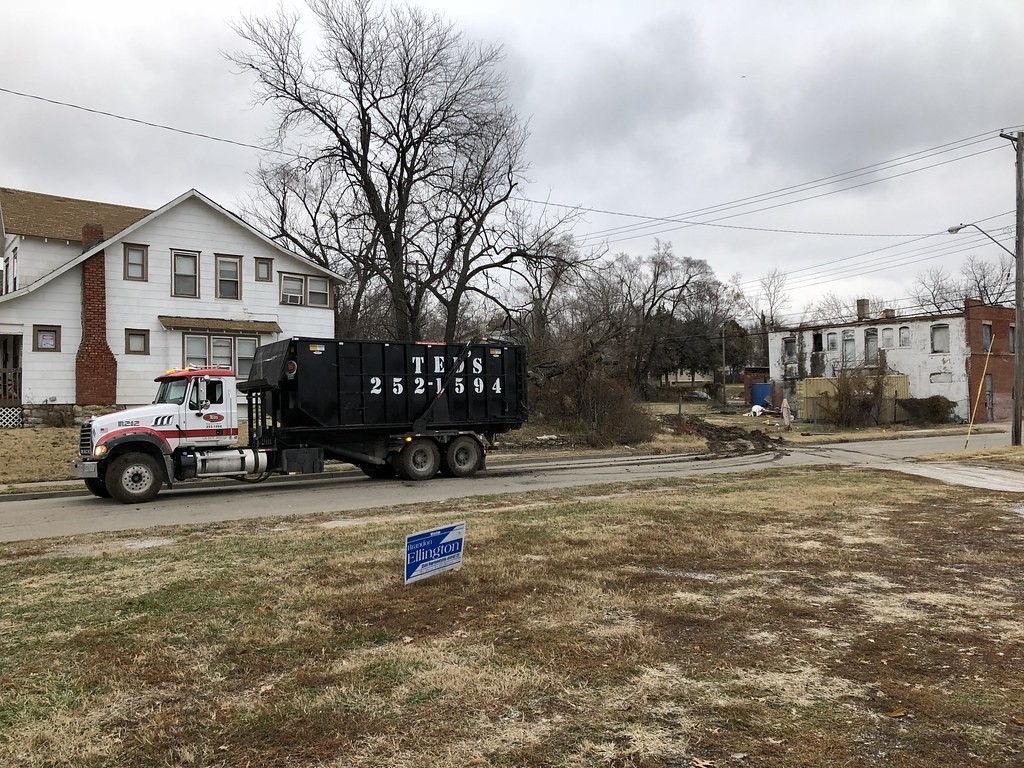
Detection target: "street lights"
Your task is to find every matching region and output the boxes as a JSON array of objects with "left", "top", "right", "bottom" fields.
[{"left": 947, "top": 223, "right": 1024, "bottom": 446}]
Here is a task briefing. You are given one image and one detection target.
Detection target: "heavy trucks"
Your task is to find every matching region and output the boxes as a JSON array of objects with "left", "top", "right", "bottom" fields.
[{"left": 70, "top": 335, "right": 529, "bottom": 504}]
